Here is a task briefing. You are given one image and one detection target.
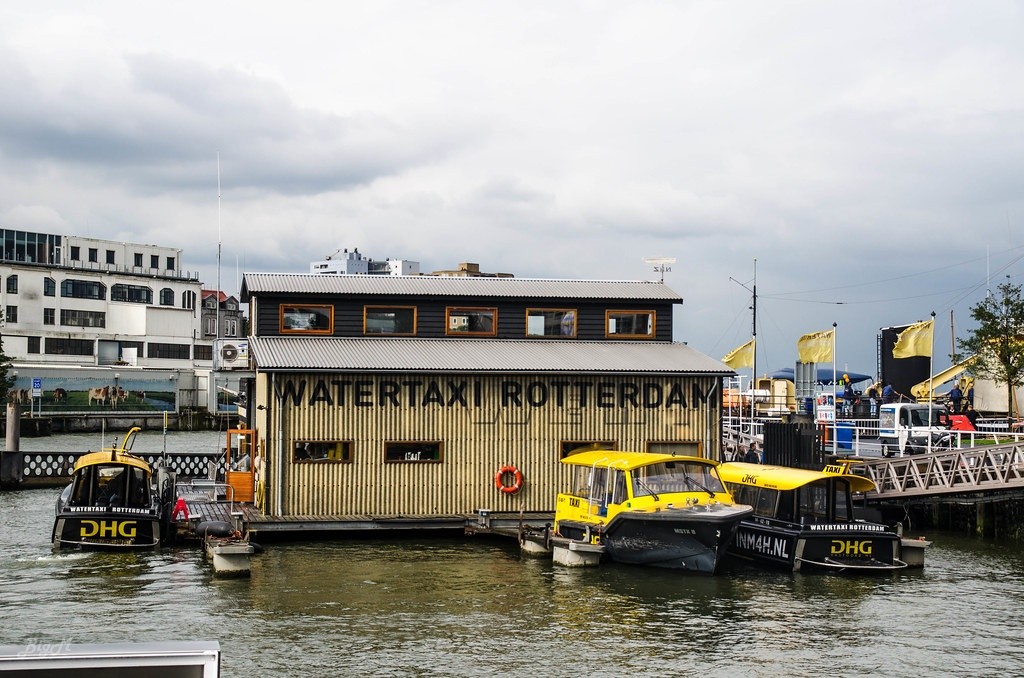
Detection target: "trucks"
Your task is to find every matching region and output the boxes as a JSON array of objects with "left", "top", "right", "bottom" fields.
[{"left": 880, "top": 404, "right": 956, "bottom": 456}]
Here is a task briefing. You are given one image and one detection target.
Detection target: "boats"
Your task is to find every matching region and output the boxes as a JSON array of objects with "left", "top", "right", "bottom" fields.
[
  {"left": 51, "top": 425, "right": 178, "bottom": 552},
  {"left": 710, "top": 462, "right": 908, "bottom": 573},
  {"left": 554, "top": 448, "right": 753, "bottom": 574}
]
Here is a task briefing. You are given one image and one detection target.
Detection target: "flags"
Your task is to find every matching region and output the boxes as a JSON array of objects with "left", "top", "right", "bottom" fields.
[
  {"left": 893, "top": 321, "right": 932, "bottom": 358},
  {"left": 722, "top": 339, "right": 754, "bottom": 368},
  {"left": 797, "top": 330, "right": 833, "bottom": 364}
]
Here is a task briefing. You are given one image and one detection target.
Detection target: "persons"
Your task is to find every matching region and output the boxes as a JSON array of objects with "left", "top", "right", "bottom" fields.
[
  {"left": 1012, "top": 421, "right": 1024, "bottom": 429},
  {"left": 735, "top": 443, "right": 759, "bottom": 464},
  {"left": 158, "top": 451, "right": 168, "bottom": 468},
  {"left": 723, "top": 445, "right": 729, "bottom": 461},
  {"left": 869, "top": 385, "right": 880, "bottom": 418},
  {"left": 945, "top": 385, "right": 963, "bottom": 413},
  {"left": 842, "top": 382, "right": 855, "bottom": 416},
  {"left": 967, "top": 382, "right": 974, "bottom": 410},
  {"left": 880, "top": 383, "right": 900, "bottom": 403},
  {"left": 295, "top": 443, "right": 325, "bottom": 460}
]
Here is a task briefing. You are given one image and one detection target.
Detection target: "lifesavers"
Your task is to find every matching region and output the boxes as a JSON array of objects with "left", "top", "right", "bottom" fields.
[{"left": 496, "top": 467, "right": 522, "bottom": 493}]
